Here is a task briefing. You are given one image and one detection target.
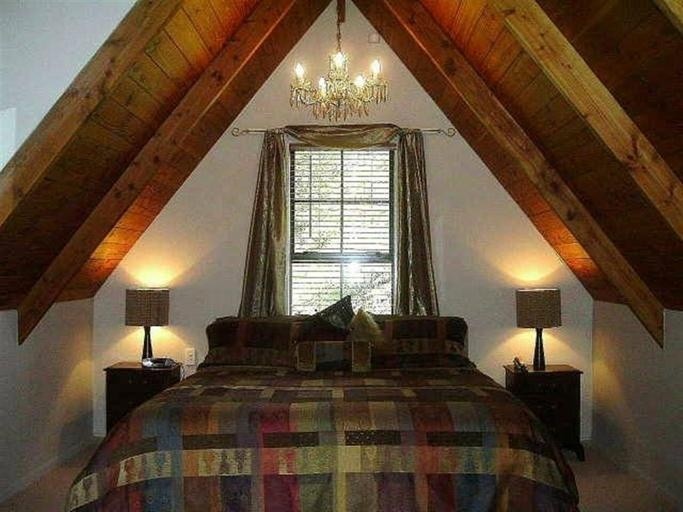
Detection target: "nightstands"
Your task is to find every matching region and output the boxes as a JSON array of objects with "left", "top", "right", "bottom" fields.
[
  {"left": 101, "top": 361, "right": 184, "bottom": 438},
  {"left": 502, "top": 365, "right": 584, "bottom": 462}
]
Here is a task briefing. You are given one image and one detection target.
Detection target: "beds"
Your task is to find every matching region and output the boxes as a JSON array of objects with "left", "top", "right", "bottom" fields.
[{"left": 62, "top": 314, "right": 581, "bottom": 512}]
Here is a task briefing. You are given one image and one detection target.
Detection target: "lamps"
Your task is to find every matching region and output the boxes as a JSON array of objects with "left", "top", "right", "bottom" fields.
[
  {"left": 515, "top": 287, "right": 563, "bottom": 372},
  {"left": 123, "top": 289, "right": 171, "bottom": 360},
  {"left": 288, "top": 0, "right": 390, "bottom": 123}
]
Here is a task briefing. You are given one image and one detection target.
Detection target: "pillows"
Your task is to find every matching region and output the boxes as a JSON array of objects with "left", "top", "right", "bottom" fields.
[{"left": 201, "top": 295, "right": 468, "bottom": 376}]
